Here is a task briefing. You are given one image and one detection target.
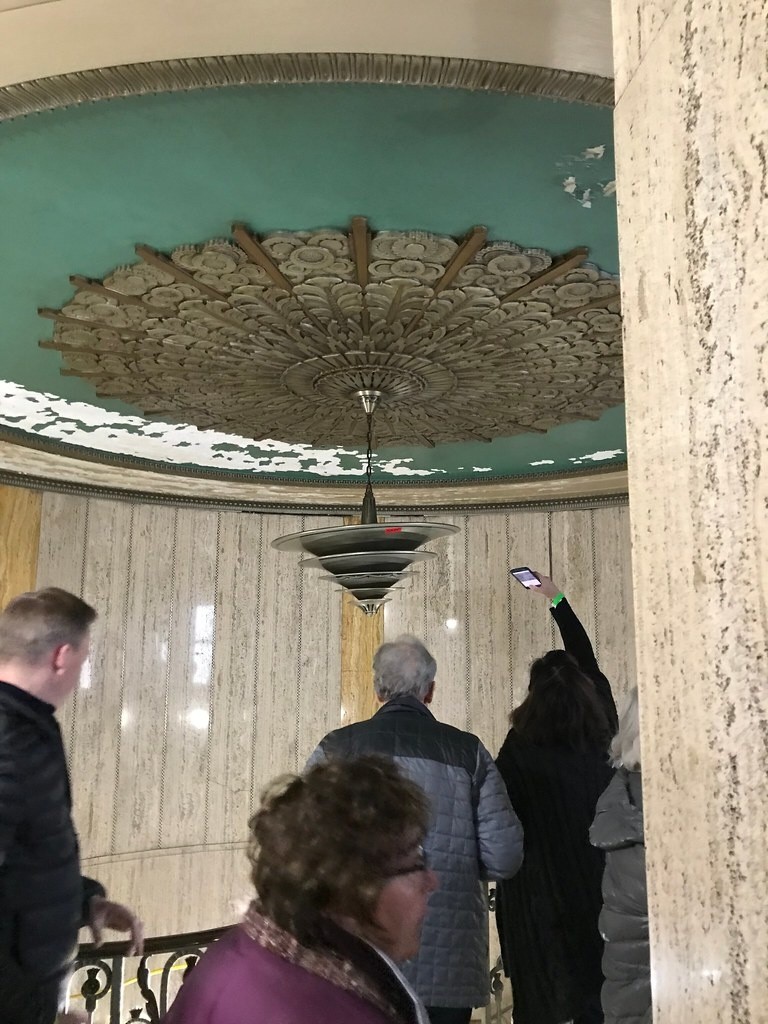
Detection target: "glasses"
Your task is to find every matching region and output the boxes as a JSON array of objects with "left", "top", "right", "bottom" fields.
[{"left": 386, "top": 845, "right": 428, "bottom": 877}]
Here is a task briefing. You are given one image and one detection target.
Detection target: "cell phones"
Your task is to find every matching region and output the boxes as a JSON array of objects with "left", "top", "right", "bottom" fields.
[{"left": 510, "top": 567, "right": 541, "bottom": 589}]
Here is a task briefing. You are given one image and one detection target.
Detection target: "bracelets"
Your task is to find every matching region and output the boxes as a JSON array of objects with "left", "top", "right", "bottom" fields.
[{"left": 551, "top": 593, "right": 563, "bottom": 608}]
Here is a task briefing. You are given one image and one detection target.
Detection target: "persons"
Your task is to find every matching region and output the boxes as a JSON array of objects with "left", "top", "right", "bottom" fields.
[
  {"left": 160, "top": 753, "right": 436, "bottom": 1024},
  {"left": 0, "top": 587, "right": 145, "bottom": 1024},
  {"left": 493, "top": 571, "right": 619, "bottom": 1024},
  {"left": 302, "top": 633, "right": 523, "bottom": 1024}
]
